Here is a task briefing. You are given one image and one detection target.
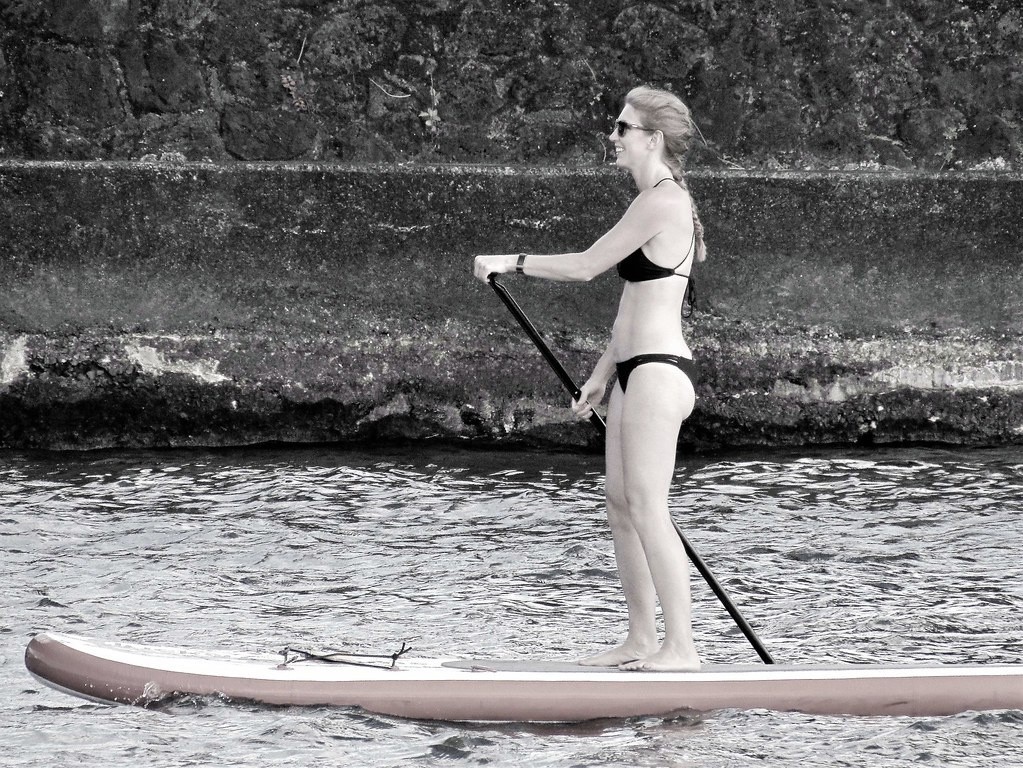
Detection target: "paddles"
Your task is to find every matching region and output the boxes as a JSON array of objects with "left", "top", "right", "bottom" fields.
[{"left": 486, "top": 269, "right": 777, "bottom": 664}]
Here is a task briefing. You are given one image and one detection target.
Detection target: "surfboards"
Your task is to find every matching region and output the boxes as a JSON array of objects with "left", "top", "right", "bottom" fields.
[{"left": 22, "top": 626, "right": 1023, "bottom": 724}]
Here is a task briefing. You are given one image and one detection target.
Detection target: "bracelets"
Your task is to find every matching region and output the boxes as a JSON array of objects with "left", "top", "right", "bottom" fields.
[{"left": 516, "top": 253, "right": 527, "bottom": 276}]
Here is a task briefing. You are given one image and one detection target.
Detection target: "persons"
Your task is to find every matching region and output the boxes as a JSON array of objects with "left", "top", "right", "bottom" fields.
[{"left": 473, "top": 86, "right": 708, "bottom": 672}]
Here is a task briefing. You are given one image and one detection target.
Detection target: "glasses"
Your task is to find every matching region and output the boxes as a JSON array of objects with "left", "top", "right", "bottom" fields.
[{"left": 614, "top": 119, "right": 657, "bottom": 136}]
